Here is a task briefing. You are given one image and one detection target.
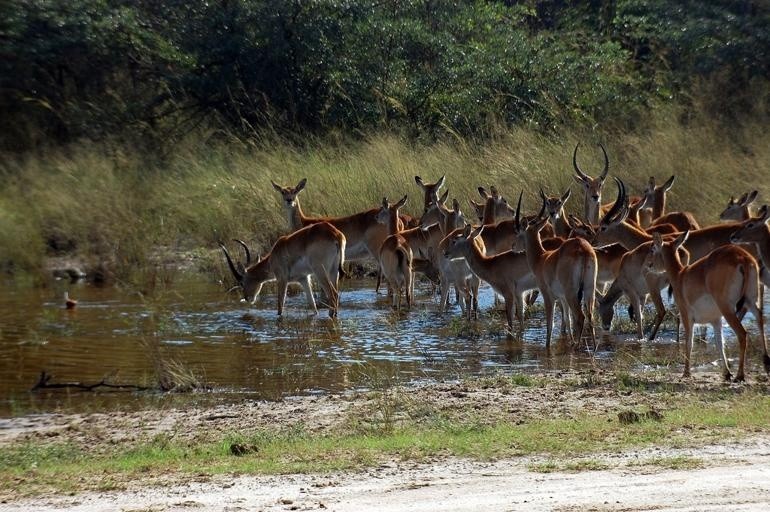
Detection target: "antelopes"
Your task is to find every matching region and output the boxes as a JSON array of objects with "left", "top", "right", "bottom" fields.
[{"left": 219, "top": 139, "right": 770, "bottom": 384}]
